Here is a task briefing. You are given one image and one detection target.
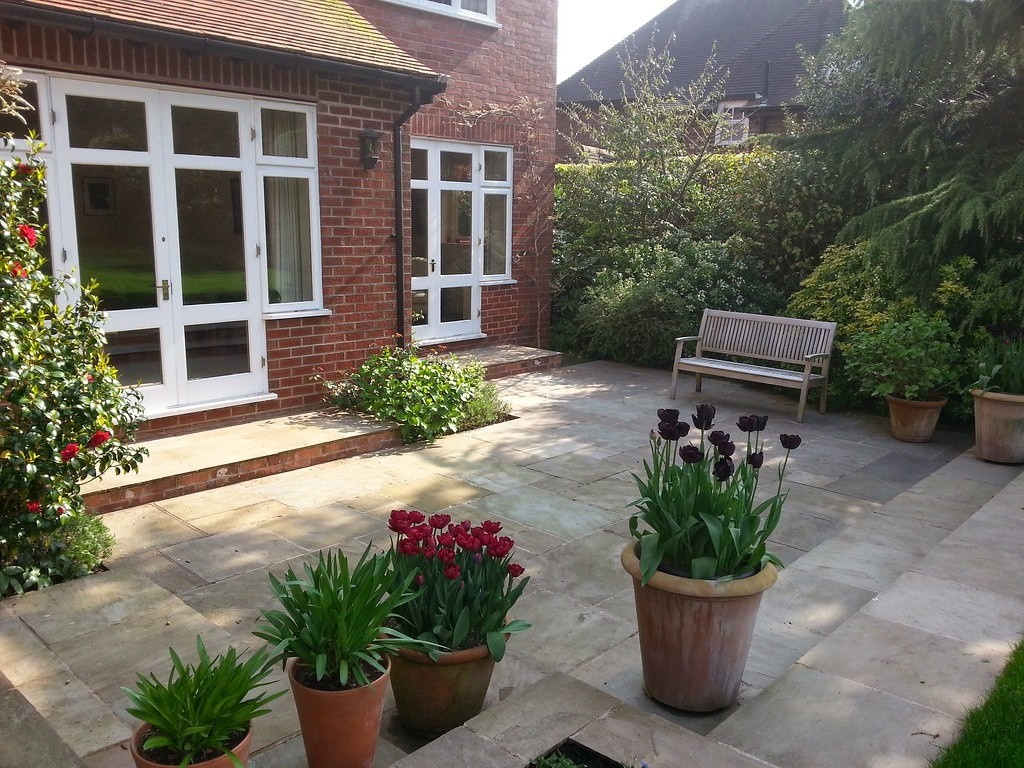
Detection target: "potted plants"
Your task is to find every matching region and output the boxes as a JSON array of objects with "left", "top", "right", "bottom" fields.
[
  {"left": 251, "top": 540, "right": 453, "bottom": 768},
  {"left": 120, "top": 633, "right": 290, "bottom": 768},
  {"left": 840, "top": 310, "right": 988, "bottom": 441},
  {"left": 969, "top": 336, "right": 1024, "bottom": 463}
]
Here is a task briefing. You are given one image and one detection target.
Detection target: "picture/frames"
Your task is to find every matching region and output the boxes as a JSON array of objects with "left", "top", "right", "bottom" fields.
[{"left": 84, "top": 179, "right": 115, "bottom": 215}]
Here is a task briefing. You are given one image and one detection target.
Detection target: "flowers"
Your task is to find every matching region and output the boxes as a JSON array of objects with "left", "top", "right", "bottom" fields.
[
  {"left": 622, "top": 404, "right": 801, "bottom": 587},
  {"left": 382, "top": 509, "right": 533, "bottom": 663}
]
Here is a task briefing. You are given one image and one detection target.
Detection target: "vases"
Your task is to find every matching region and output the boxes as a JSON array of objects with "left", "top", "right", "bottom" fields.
[
  {"left": 620, "top": 536, "right": 779, "bottom": 713},
  {"left": 377, "top": 615, "right": 512, "bottom": 740}
]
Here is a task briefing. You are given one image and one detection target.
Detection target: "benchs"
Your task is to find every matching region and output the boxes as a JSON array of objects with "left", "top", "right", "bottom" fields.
[{"left": 670, "top": 308, "right": 837, "bottom": 423}]
